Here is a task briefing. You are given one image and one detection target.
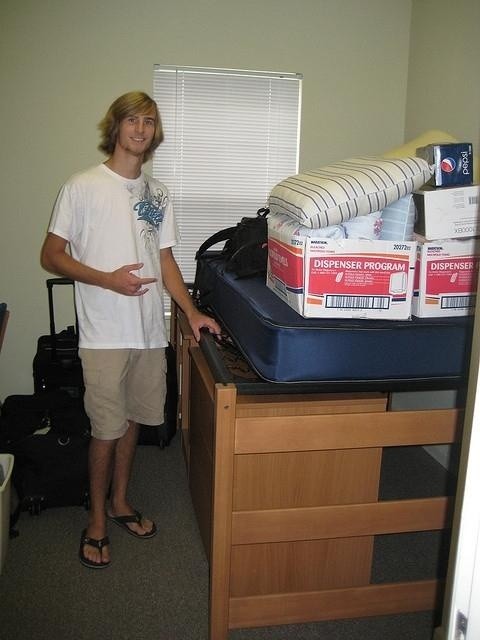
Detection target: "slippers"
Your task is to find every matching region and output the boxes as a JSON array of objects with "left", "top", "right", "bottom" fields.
[
  {"left": 103, "top": 505, "right": 160, "bottom": 540},
  {"left": 77, "top": 527, "right": 112, "bottom": 570}
]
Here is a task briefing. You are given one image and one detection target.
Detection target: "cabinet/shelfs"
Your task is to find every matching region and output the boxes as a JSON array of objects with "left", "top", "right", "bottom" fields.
[{"left": 164, "top": 286, "right": 474, "bottom": 640}]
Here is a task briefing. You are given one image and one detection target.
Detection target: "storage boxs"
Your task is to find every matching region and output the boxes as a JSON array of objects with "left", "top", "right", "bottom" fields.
[{"left": 266, "top": 142, "right": 480, "bottom": 321}]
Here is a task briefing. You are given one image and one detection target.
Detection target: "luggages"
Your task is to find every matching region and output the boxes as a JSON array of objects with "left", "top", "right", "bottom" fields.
[
  {"left": 135, "top": 339, "right": 177, "bottom": 449},
  {"left": 32, "top": 275, "right": 90, "bottom": 442}
]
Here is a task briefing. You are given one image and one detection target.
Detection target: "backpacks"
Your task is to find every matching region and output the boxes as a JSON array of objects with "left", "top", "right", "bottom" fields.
[
  {"left": 0, "top": 394, "right": 49, "bottom": 453},
  {"left": 3, "top": 428, "right": 93, "bottom": 515}
]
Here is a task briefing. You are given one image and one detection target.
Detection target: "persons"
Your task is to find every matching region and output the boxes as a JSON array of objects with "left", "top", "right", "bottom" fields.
[{"left": 38, "top": 90, "right": 224, "bottom": 570}]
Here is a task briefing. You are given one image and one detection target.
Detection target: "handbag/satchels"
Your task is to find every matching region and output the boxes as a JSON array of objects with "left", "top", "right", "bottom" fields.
[{"left": 222, "top": 216, "right": 269, "bottom": 275}]
